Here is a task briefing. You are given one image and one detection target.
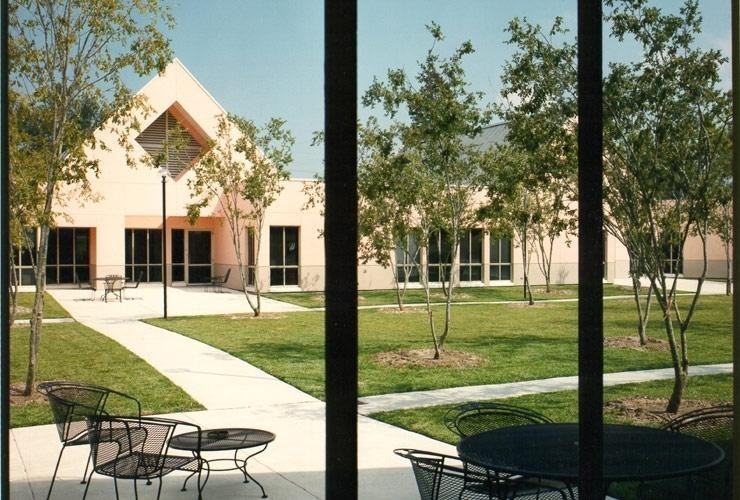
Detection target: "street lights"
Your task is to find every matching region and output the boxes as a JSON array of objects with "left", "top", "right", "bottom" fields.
[{"left": 157, "top": 168, "right": 171, "bottom": 319}]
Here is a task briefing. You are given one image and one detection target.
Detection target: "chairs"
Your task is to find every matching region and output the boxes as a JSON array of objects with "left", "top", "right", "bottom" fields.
[
  {"left": 75, "top": 271, "right": 144, "bottom": 303},
  {"left": 36, "top": 381, "right": 201, "bottom": 500},
  {"left": 204, "top": 269, "right": 232, "bottom": 293}
]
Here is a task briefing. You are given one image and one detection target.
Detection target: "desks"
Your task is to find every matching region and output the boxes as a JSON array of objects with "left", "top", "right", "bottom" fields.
[{"left": 168, "top": 428, "right": 276, "bottom": 500}]
